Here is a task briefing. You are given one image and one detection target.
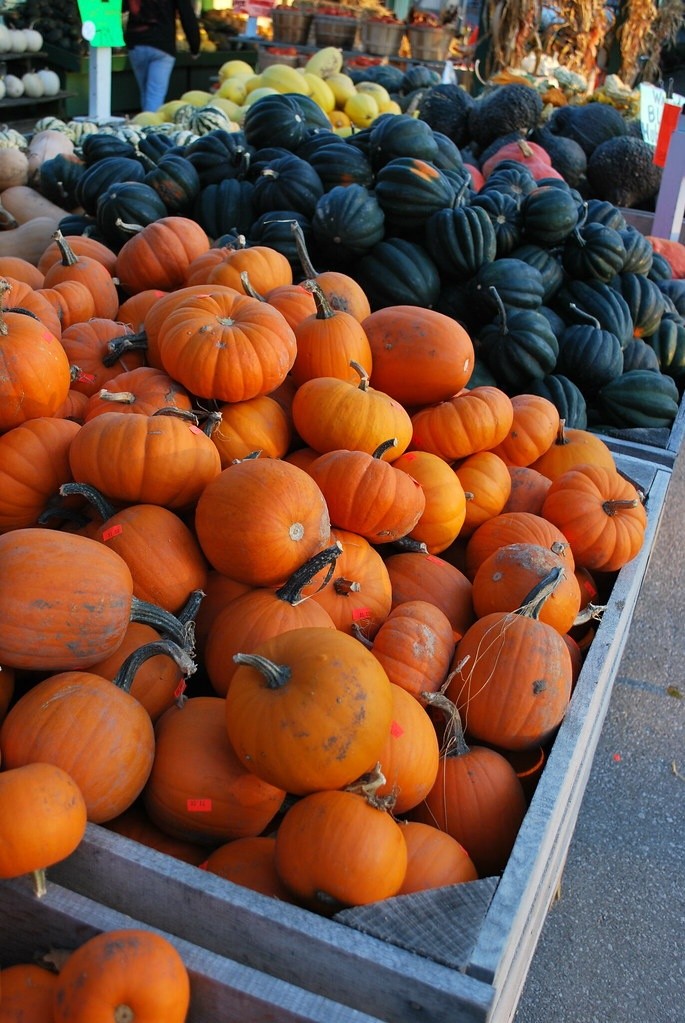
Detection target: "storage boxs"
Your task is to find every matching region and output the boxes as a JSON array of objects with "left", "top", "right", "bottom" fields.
[{"left": 1, "top": 397, "right": 684, "bottom": 1023}]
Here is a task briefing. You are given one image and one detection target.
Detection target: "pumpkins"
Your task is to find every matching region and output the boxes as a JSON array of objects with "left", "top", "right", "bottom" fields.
[{"left": 0, "top": 0, "right": 685, "bottom": 1023}]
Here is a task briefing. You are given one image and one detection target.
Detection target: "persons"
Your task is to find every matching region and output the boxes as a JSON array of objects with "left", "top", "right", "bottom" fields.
[{"left": 120, "top": 0, "right": 202, "bottom": 115}]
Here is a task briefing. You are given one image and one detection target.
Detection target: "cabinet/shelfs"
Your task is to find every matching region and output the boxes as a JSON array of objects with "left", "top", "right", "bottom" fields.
[{"left": 0, "top": 51, "right": 78, "bottom": 134}]
